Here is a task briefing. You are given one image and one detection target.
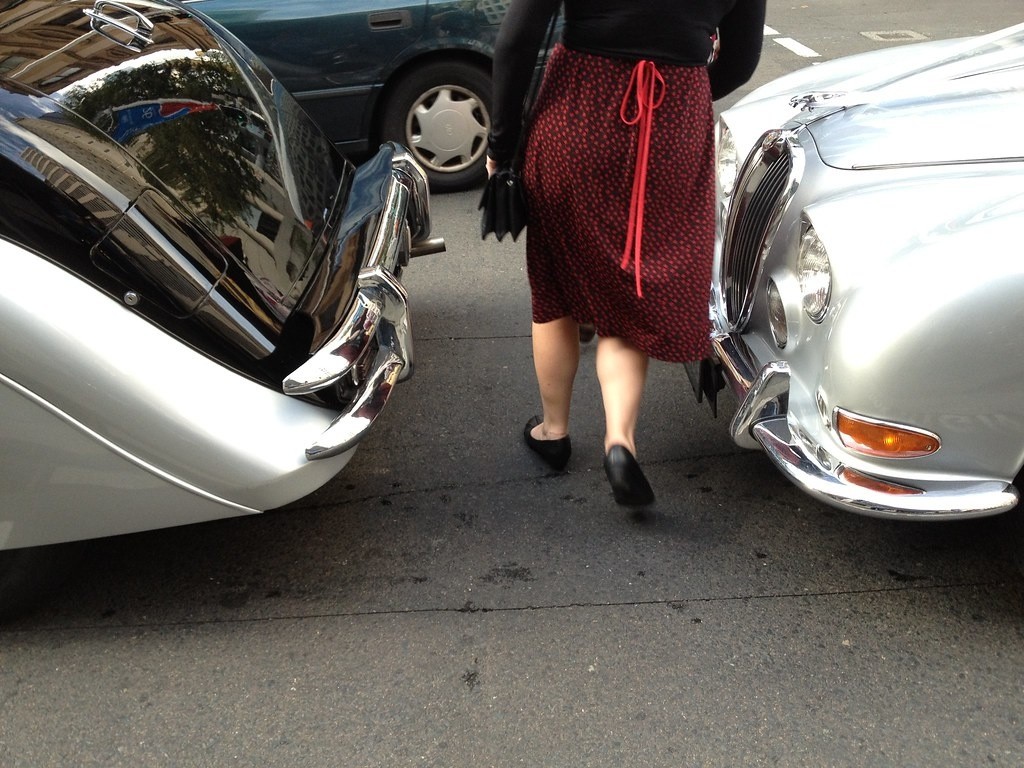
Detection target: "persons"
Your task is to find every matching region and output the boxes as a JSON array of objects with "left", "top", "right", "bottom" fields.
[{"left": 485, "top": 0, "right": 767, "bottom": 505}]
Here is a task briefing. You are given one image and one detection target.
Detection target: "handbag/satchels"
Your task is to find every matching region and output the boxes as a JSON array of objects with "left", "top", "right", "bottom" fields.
[{"left": 478, "top": 118, "right": 530, "bottom": 242}]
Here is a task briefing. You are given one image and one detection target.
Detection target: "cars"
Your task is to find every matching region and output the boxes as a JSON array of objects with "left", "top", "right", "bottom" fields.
[
  {"left": 0, "top": 0, "right": 447, "bottom": 549},
  {"left": 683, "top": 21, "right": 1024, "bottom": 523},
  {"left": 186, "top": 0, "right": 564, "bottom": 189}
]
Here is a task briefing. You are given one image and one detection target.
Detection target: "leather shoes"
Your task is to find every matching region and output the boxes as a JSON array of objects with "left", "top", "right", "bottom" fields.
[
  {"left": 604, "top": 445, "right": 655, "bottom": 507},
  {"left": 524, "top": 414, "right": 571, "bottom": 470}
]
